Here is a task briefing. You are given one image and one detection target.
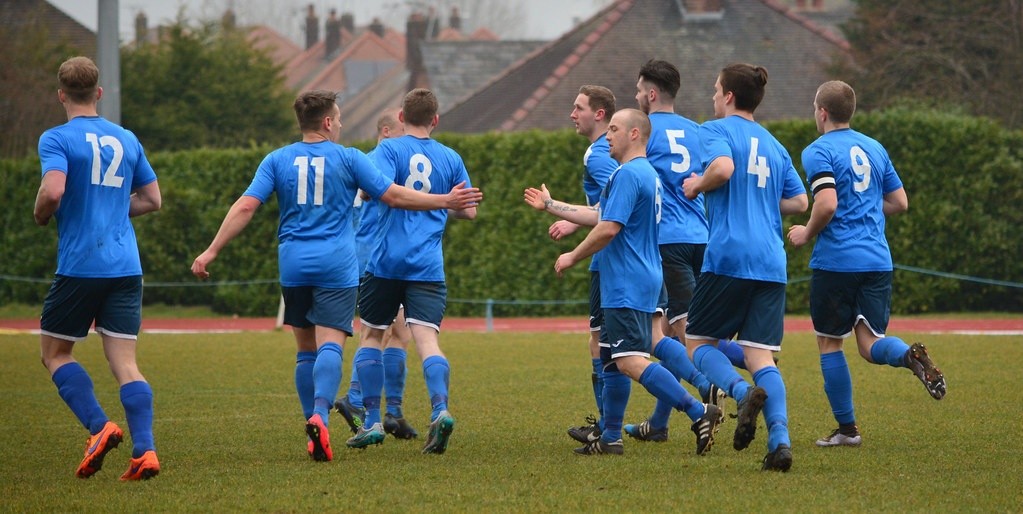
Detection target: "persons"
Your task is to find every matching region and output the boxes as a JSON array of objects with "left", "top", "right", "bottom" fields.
[
  {"left": 191, "top": 89, "right": 482, "bottom": 464},
  {"left": 33, "top": 57, "right": 162, "bottom": 480},
  {"left": 786, "top": 81, "right": 947, "bottom": 445},
  {"left": 549, "top": 85, "right": 726, "bottom": 445},
  {"left": 678, "top": 62, "right": 809, "bottom": 468},
  {"left": 524, "top": 107, "right": 720, "bottom": 456},
  {"left": 622, "top": 60, "right": 778, "bottom": 443},
  {"left": 333, "top": 89, "right": 477, "bottom": 454}
]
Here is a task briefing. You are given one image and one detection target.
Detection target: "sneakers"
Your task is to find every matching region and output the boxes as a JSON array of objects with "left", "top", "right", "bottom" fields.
[
  {"left": 904, "top": 342, "right": 947, "bottom": 399},
  {"left": 814, "top": 423, "right": 861, "bottom": 447},
  {"left": 567, "top": 413, "right": 604, "bottom": 445},
  {"left": 383, "top": 413, "right": 418, "bottom": 440},
  {"left": 334, "top": 395, "right": 367, "bottom": 434},
  {"left": 118, "top": 451, "right": 160, "bottom": 482},
  {"left": 624, "top": 416, "right": 668, "bottom": 443},
  {"left": 572, "top": 435, "right": 624, "bottom": 456},
  {"left": 304, "top": 413, "right": 333, "bottom": 461},
  {"left": 772, "top": 355, "right": 779, "bottom": 365},
  {"left": 75, "top": 421, "right": 123, "bottom": 478},
  {"left": 421, "top": 410, "right": 454, "bottom": 454},
  {"left": 702, "top": 382, "right": 729, "bottom": 427},
  {"left": 344, "top": 422, "right": 385, "bottom": 449},
  {"left": 690, "top": 402, "right": 723, "bottom": 456},
  {"left": 728, "top": 387, "right": 768, "bottom": 451},
  {"left": 761, "top": 443, "right": 793, "bottom": 471}
]
